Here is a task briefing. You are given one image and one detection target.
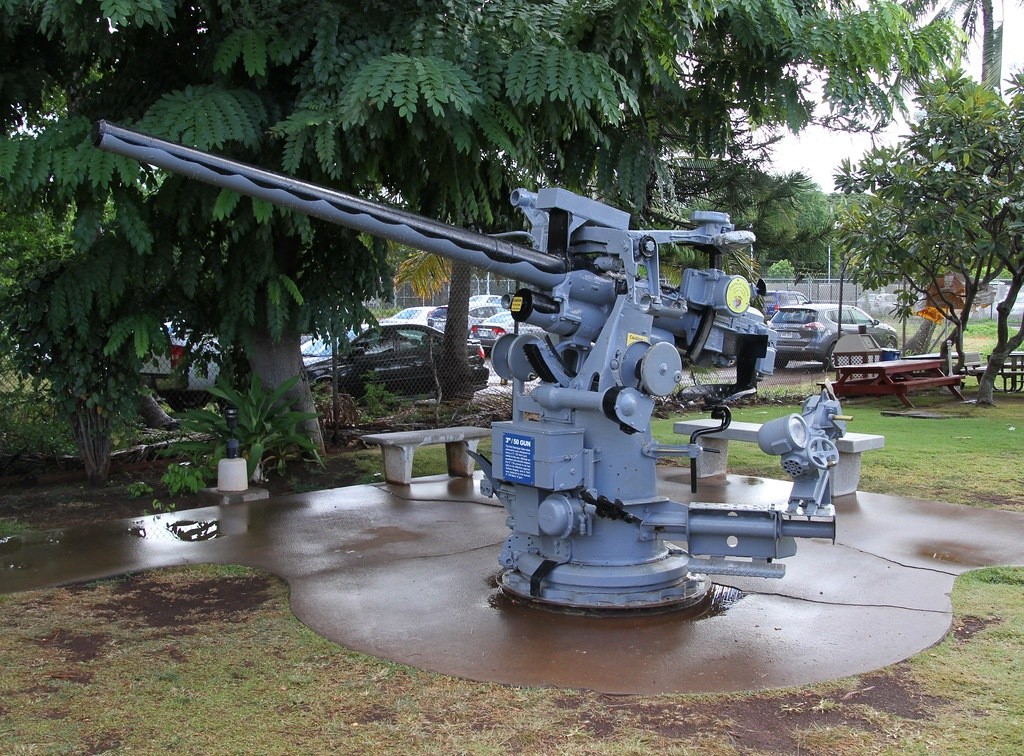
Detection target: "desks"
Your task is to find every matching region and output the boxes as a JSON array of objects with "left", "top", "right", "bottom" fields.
[
  {"left": 832, "top": 359, "right": 966, "bottom": 408},
  {"left": 987, "top": 350, "right": 1024, "bottom": 393},
  {"left": 901, "top": 352, "right": 979, "bottom": 360}
]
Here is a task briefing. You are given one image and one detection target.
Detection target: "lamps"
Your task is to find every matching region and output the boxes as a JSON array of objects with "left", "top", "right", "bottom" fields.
[{"left": 225, "top": 406, "right": 238, "bottom": 430}]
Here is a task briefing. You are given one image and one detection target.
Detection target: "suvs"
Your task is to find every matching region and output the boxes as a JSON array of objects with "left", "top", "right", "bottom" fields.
[{"left": 766, "top": 304, "right": 899, "bottom": 371}]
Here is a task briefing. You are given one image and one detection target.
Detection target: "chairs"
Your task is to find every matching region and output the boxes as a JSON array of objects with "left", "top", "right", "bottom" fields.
[{"left": 676, "top": 333, "right": 768, "bottom": 493}]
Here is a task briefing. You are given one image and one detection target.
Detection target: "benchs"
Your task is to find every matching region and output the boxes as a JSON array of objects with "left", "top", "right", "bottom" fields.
[
  {"left": 673, "top": 418, "right": 885, "bottom": 497},
  {"left": 816, "top": 376, "right": 878, "bottom": 386},
  {"left": 959, "top": 362, "right": 1024, "bottom": 392},
  {"left": 362, "top": 426, "right": 493, "bottom": 484},
  {"left": 894, "top": 374, "right": 965, "bottom": 394}
]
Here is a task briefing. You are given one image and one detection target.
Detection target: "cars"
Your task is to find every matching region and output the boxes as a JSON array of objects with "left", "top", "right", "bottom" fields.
[
  {"left": 137, "top": 313, "right": 316, "bottom": 414},
  {"left": 379, "top": 305, "right": 482, "bottom": 339},
  {"left": 471, "top": 311, "right": 549, "bottom": 348},
  {"left": 468, "top": 294, "right": 510, "bottom": 326},
  {"left": 298, "top": 319, "right": 489, "bottom": 403},
  {"left": 763, "top": 290, "right": 810, "bottom": 321}
]
